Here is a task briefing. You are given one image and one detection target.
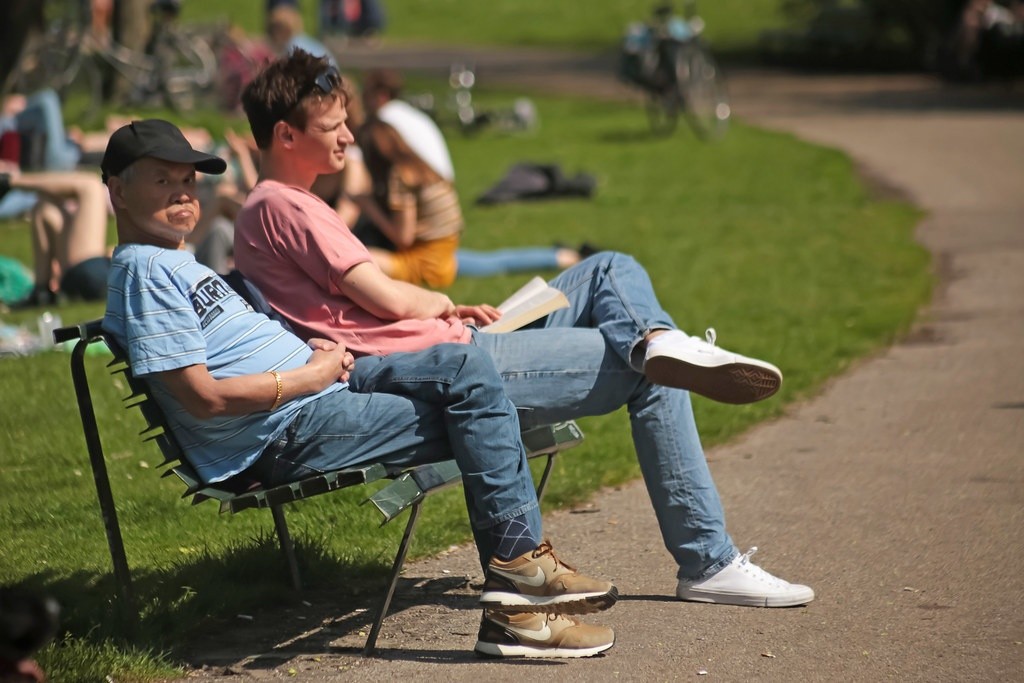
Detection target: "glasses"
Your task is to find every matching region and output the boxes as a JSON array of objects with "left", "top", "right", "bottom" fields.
[{"left": 277, "top": 66, "right": 342, "bottom": 120}]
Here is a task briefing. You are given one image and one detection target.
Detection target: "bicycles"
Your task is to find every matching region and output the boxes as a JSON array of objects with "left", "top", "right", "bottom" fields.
[
  {"left": 22, "top": 0, "right": 218, "bottom": 131},
  {"left": 637, "top": 3, "right": 729, "bottom": 143}
]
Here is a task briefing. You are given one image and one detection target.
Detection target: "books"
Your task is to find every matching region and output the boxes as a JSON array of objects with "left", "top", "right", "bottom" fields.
[{"left": 478, "top": 275, "right": 571, "bottom": 332}]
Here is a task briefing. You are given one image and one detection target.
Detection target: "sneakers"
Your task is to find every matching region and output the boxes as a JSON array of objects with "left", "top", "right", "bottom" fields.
[
  {"left": 676, "top": 547, "right": 815, "bottom": 607},
  {"left": 479, "top": 539, "right": 618, "bottom": 614},
  {"left": 474, "top": 608, "right": 616, "bottom": 658},
  {"left": 643, "top": 327, "right": 783, "bottom": 404}
]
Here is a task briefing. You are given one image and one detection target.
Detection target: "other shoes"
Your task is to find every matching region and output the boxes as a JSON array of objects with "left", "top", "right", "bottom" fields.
[{"left": 31, "top": 284, "right": 58, "bottom": 305}]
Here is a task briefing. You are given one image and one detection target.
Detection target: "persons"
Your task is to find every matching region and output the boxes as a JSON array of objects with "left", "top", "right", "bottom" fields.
[
  {"left": 234, "top": 46, "right": 816, "bottom": 608},
  {"left": 102, "top": 119, "right": 619, "bottom": 657},
  {"left": 0, "top": 1, "right": 600, "bottom": 298}
]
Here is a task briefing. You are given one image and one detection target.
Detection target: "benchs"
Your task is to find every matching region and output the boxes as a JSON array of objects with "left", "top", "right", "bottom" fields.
[{"left": 51, "top": 315, "right": 585, "bottom": 657}]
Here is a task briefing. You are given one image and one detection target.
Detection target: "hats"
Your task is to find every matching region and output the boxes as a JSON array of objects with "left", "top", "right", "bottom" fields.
[{"left": 101, "top": 119, "right": 227, "bottom": 182}]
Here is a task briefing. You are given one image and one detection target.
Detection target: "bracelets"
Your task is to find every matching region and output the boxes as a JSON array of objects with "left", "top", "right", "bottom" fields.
[{"left": 270, "top": 370, "right": 282, "bottom": 412}]
